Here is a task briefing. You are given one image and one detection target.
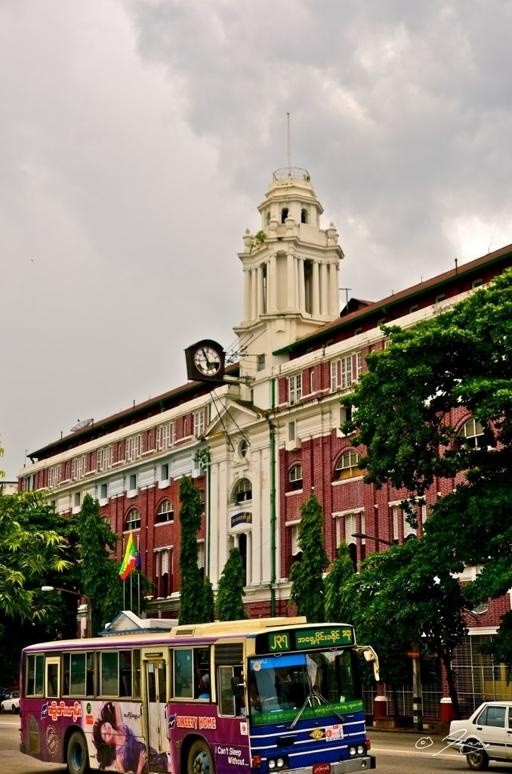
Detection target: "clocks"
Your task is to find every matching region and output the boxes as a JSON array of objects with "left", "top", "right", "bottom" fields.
[{"left": 183, "top": 339, "right": 226, "bottom": 382}]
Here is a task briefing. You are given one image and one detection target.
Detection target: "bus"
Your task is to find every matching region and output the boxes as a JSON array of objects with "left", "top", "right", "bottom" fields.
[{"left": 19, "top": 616, "right": 380, "bottom": 774}]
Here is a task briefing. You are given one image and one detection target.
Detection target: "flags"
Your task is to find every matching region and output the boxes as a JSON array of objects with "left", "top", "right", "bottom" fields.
[{"left": 118, "top": 526, "right": 141, "bottom": 580}]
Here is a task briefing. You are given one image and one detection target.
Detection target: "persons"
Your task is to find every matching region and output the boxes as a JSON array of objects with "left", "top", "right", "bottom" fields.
[
  {"left": 197, "top": 671, "right": 209, "bottom": 689},
  {"left": 92, "top": 702, "right": 168, "bottom": 774}
]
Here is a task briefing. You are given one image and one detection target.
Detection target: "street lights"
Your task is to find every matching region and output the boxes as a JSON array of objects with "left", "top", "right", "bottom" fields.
[{"left": 41, "top": 586, "right": 92, "bottom": 639}]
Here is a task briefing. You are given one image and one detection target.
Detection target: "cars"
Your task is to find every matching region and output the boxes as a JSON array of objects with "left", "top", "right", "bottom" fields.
[
  {"left": 447, "top": 701, "right": 512, "bottom": 770},
  {"left": 1, "top": 691, "right": 19, "bottom": 714}
]
